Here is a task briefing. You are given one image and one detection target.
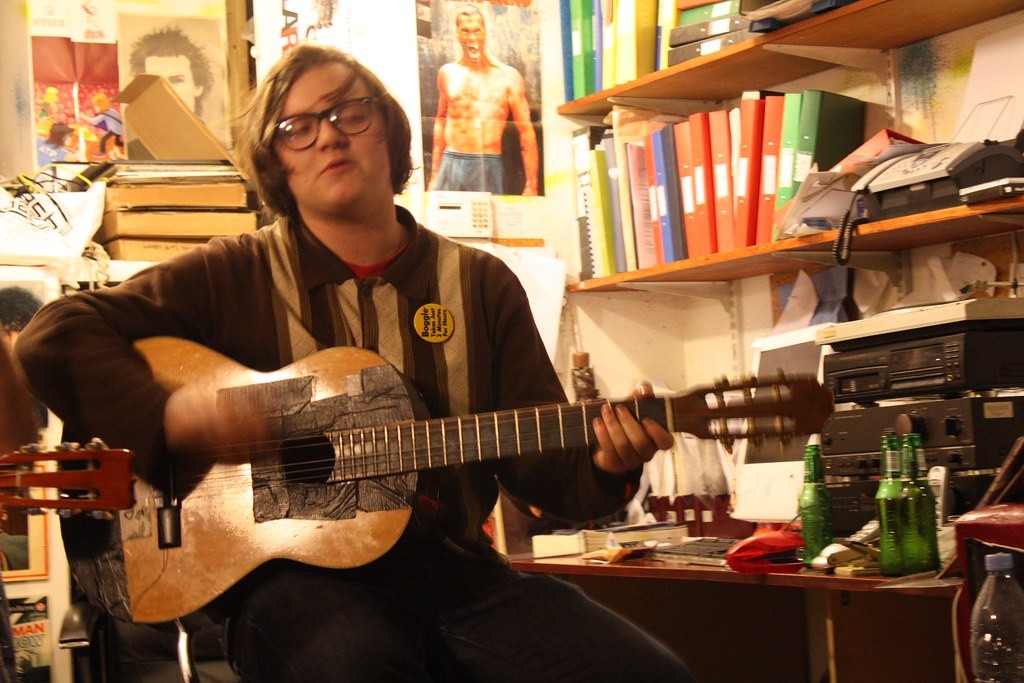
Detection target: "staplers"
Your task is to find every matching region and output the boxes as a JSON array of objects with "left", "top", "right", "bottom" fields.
[{"left": 728, "top": 531, "right": 805, "bottom": 573}]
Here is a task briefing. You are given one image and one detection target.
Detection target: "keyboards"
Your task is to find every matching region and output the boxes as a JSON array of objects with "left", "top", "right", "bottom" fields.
[{"left": 648, "top": 537, "right": 743, "bottom": 566}]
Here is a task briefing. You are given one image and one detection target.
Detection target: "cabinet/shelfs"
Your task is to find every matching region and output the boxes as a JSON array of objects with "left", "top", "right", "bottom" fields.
[{"left": 558, "top": 0, "right": 1024, "bottom": 376}]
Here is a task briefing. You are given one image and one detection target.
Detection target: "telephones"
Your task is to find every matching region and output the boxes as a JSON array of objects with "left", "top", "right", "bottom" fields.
[
  {"left": 926, "top": 466, "right": 948, "bottom": 526},
  {"left": 810, "top": 520, "right": 879, "bottom": 574},
  {"left": 833, "top": 142, "right": 1024, "bottom": 222}
]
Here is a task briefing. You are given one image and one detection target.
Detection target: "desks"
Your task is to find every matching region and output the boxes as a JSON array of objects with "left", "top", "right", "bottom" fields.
[{"left": 507, "top": 538, "right": 970, "bottom": 683}]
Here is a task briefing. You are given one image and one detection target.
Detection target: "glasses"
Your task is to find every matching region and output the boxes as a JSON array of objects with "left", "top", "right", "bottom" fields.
[{"left": 274, "top": 97, "right": 381, "bottom": 152}]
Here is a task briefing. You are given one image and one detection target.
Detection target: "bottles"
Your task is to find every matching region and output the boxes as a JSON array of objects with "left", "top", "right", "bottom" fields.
[
  {"left": 900, "top": 434, "right": 925, "bottom": 573},
  {"left": 969, "top": 554, "right": 1024, "bottom": 683},
  {"left": 798, "top": 444, "right": 832, "bottom": 566},
  {"left": 911, "top": 435, "right": 936, "bottom": 569},
  {"left": 877, "top": 432, "right": 903, "bottom": 573}
]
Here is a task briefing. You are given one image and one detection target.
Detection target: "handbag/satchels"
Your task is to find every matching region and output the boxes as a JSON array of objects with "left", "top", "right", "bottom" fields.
[{"left": 0, "top": 164, "right": 108, "bottom": 267}]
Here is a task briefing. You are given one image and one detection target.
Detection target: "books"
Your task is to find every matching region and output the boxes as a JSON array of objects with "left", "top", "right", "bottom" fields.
[{"left": 532, "top": 523, "right": 689, "bottom": 560}]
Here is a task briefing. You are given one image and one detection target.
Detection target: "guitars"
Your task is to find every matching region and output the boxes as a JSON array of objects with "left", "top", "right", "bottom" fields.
[
  {"left": 0, "top": 437, "right": 138, "bottom": 531},
  {"left": 55, "top": 333, "right": 839, "bottom": 628}
]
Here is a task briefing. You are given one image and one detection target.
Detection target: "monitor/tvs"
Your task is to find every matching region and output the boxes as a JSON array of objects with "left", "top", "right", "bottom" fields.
[{"left": 728, "top": 322, "right": 836, "bottom": 525}]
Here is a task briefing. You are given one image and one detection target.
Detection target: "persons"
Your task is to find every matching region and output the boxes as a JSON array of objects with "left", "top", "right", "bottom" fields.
[
  {"left": 423, "top": 8, "right": 541, "bottom": 194},
  {"left": 12, "top": 42, "right": 692, "bottom": 683},
  {"left": 0, "top": 285, "right": 48, "bottom": 683}
]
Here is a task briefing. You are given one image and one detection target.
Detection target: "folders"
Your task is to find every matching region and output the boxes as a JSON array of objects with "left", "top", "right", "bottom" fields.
[
  {"left": 559, "top": 1, "right": 758, "bottom": 105},
  {"left": 571, "top": 90, "right": 867, "bottom": 282}
]
